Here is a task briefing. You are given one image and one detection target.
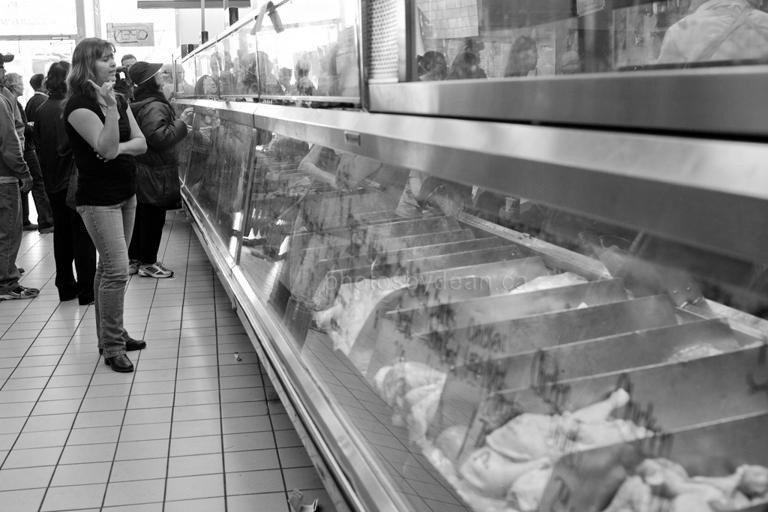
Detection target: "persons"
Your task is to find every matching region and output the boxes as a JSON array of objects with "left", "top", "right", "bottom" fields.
[{"left": 57, "top": 37, "right": 147, "bottom": 373}]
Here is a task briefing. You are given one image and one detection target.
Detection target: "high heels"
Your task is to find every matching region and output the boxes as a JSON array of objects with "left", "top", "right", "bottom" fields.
[
  {"left": 98, "top": 337, "right": 148, "bottom": 356},
  {"left": 103, "top": 352, "right": 135, "bottom": 374}
]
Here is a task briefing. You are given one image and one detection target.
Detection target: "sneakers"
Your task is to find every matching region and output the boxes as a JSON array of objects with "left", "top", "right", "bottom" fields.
[
  {"left": 137, "top": 261, "right": 175, "bottom": 279},
  {"left": 127, "top": 258, "right": 141, "bottom": 276},
  {"left": 0, "top": 286, "right": 40, "bottom": 301},
  {"left": 17, "top": 267, "right": 26, "bottom": 275}
]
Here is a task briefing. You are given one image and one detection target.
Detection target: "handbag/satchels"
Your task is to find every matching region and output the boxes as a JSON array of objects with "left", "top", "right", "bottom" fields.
[{"left": 133, "top": 160, "right": 183, "bottom": 213}]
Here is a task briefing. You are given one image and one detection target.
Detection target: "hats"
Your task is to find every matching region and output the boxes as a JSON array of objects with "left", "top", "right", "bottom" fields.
[
  {"left": 0, "top": 53, "right": 14, "bottom": 63},
  {"left": 128, "top": 61, "right": 164, "bottom": 86}
]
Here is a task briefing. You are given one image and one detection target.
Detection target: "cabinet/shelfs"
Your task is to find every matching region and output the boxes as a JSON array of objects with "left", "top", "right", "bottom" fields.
[
  {"left": 231, "top": 130, "right": 765, "bottom": 510},
  {"left": 364, "top": 2, "right": 767, "bottom": 138},
  {"left": 175, "top": 102, "right": 254, "bottom": 311},
  {"left": 172, "top": 13, "right": 257, "bottom": 98},
  {"left": 257, "top": 1, "right": 361, "bottom": 103}
]
